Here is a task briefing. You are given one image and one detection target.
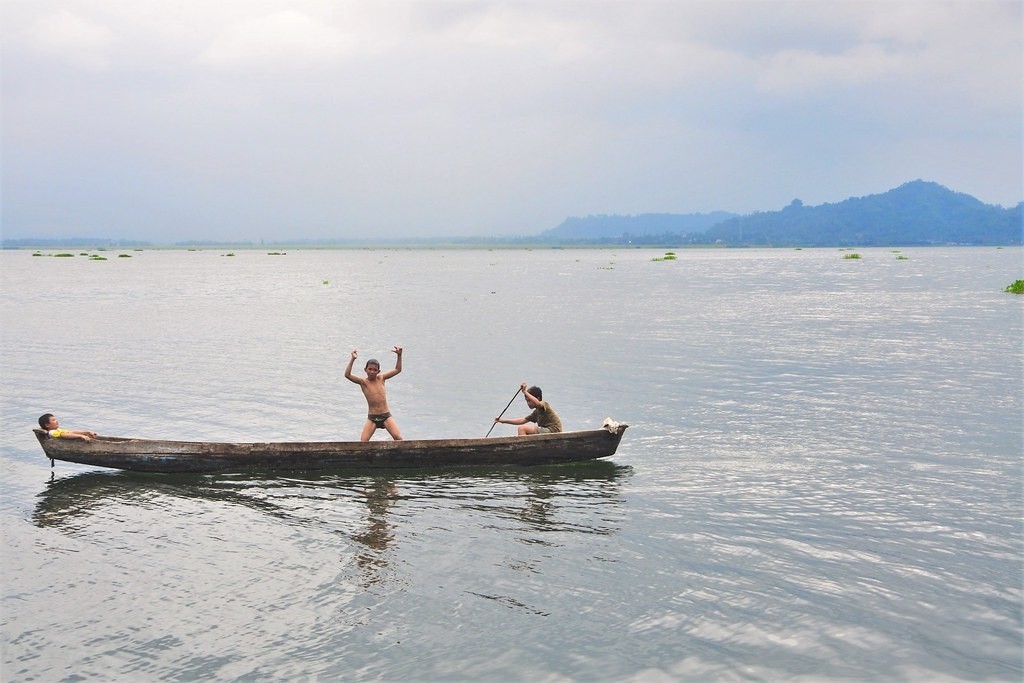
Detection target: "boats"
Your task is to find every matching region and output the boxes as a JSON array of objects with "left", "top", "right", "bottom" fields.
[{"left": 32, "top": 425, "right": 631, "bottom": 475}]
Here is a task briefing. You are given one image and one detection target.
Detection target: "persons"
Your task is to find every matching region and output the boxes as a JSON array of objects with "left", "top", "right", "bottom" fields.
[
  {"left": 494, "top": 383, "right": 562, "bottom": 436},
  {"left": 345, "top": 344, "right": 404, "bottom": 441},
  {"left": 38, "top": 413, "right": 139, "bottom": 443}
]
